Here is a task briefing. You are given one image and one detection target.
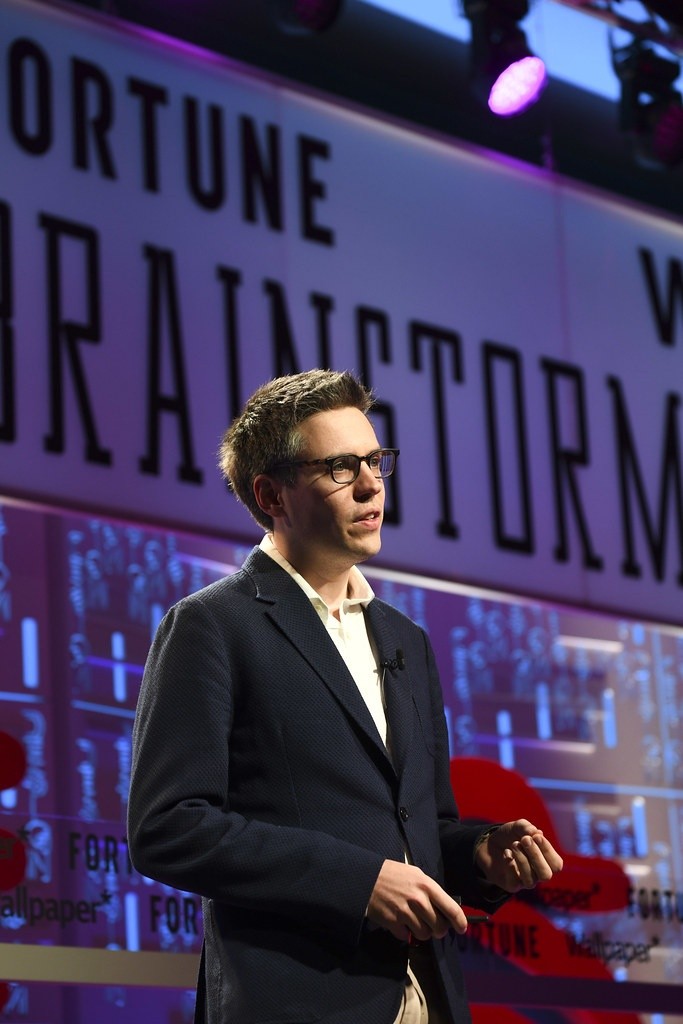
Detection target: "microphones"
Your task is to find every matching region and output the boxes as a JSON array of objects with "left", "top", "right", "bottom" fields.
[{"left": 381, "top": 648, "right": 405, "bottom": 670}]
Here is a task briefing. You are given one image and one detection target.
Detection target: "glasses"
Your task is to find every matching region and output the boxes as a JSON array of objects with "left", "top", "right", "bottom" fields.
[{"left": 266, "top": 449, "right": 402, "bottom": 485}]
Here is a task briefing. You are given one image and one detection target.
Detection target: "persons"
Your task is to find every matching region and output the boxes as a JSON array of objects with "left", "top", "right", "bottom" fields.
[{"left": 125, "top": 367, "right": 567, "bottom": 1024}]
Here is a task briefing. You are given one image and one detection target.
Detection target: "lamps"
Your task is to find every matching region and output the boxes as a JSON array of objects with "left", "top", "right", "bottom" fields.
[{"left": 461, "top": 1, "right": 548, "bottom": 121}]
